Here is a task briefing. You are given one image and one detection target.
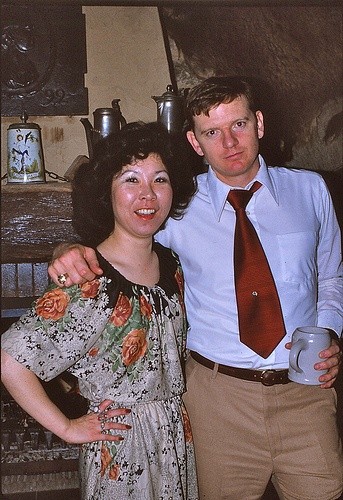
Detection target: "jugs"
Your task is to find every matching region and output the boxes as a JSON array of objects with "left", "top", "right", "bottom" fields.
[
  {"left": 80, "top": 97, "right": 127, "bottom": 159},
  {"left": 151, "top": 85, "right": 187, "bottom": 134}
]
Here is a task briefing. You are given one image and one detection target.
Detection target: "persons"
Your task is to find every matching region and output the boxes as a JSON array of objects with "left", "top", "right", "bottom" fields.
[
  {"left": 45, "top": 75, "right": 343, "bottom": 500},
  {"left": 0, "top": 121, "right": 201, "bottom": 500}
]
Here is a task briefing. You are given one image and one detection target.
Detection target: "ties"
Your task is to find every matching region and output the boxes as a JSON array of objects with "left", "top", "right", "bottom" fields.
[{"left": 226, "top": 180, "right": 287, "bottom": 359}]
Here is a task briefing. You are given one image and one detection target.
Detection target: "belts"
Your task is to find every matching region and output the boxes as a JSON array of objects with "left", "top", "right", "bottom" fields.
[{"left": 190, "top": 350, "right": 292, "bottom": 386}]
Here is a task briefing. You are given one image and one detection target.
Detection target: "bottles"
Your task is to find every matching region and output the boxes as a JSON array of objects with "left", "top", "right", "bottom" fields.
[{"left": 7, "top": 111, "right": 46, "bottom": 184}]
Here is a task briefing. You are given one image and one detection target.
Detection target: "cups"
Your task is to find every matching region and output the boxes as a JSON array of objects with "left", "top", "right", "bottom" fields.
[{"left": 288, "top": 327, "right": 331, "bottom": 386}]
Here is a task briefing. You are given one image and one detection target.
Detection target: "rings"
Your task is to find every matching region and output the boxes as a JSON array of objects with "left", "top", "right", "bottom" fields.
[{"left": 57, "top": 272, "right": 68, "bottom": 285}]
